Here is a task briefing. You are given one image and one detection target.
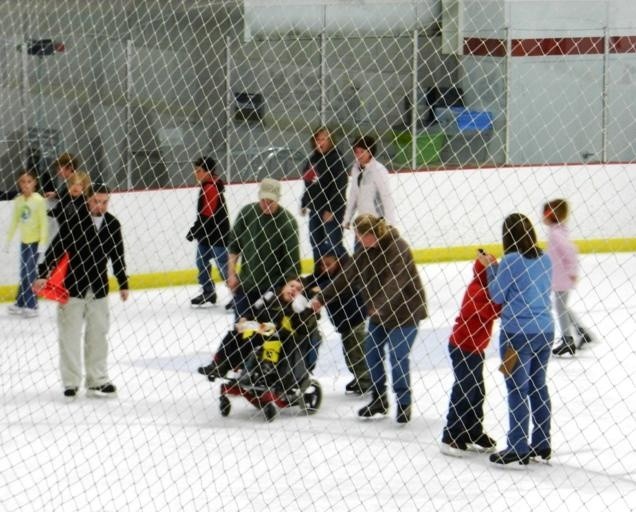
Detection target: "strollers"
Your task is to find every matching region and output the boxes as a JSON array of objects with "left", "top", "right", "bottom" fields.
[{"left": 218, "top": 295, "right": 324, "bottom": 417}]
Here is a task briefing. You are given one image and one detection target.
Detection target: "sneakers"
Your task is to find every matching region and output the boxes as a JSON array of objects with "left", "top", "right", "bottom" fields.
[
  {"left": 490, "top": 448, "right": 528, "bottom": 464},
  {"left": 63, "top": 386, "right": 78, "bottom": 397},
  {"left": 21, "top": 307, "right": 36, "bottom": 314},
  {"left": 197, "top": 362, "right": 225, "bottom": 376},
  {"left": 577, "top": 327, "right": 591, "bottom": 348},
  {"left": 528, "top": 448, "right": 552, "bottom": 460},
  {"left": 191, "top": 291, "right": 217, "bottom": 303},
  {"left": 347, "top": 376, "right": 358, "bottom": 391},
  {"left": 89, "top": 383, "right": 116, "bottom": 394},
  {"left": 441, "top": 427, "right": 469, "bottom": 450},
  {"left": 397, "top": 403, "right": 411, "bottom": 423},
  {"left": 552, "top": 336, "right": 575, "bottom": 354},
  {"left": 359, "top": 386, "right": 388, "bottom": 417},
  {"left": 462, "top": 428, "right": 496, "bottom": 447},
  {"left": 8, "top": 304, "right": 21, "bottom": 312}
]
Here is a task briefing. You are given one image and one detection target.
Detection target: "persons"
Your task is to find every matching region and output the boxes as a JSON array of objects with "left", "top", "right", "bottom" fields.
[
  {"left": 28, "top": 183, "right": 130, "bottom": 399},
  {"left": 195, "top": 276, "right": 321, "bottom": 395},
  {"left": 540, "top": 197, "right": 595, "bottom": 359},
  {"left": 225, "top": 178, "right": 300, "bottom": 323},
  {"left": 439, "top": 251, "right": 503, "bottom": 451},
  {"left": 46, "top": 168, "right": 95, "bottom": 229},
  {"left": 291, "top": 245, "right": 370, "bottom": 395},
  {"left": 307, "top": 211, "right": 428, "bottom": 424},
  {"left": 2, "top": 166, "right": 50, "bottom": 318},
  {"left": 340, "top": 133, "right": 398, "bottom": 255},
  {"left": 299, "top": 125, "right": 350, "bottom": 302},
  {"left": 41, "top": 152, "right": 79, "bottom": 224},
  {"left": 476, "top": 212, "right": 554, "bottom": 466},
  {"left": 185, "top": 154, "right": 234, "bottom": 306},
  {"left": 0, "top": 147, "right": 58, "bottom": 200}
]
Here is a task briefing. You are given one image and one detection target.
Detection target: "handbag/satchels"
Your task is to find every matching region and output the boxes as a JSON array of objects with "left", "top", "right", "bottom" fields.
[{"left": 498, "top": 348, "right": 517, "bottom": 374}]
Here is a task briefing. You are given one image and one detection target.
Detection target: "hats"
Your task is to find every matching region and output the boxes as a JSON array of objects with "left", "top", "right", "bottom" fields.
[
  {"left": 544, "top": 199, "right": 567, "bottom": 222},
  {"left": 473, "top": 255, "right": 497, "bottom": 279},
  {"left": 258, "top": 178, "right": 282, "bottom": 203}
]
